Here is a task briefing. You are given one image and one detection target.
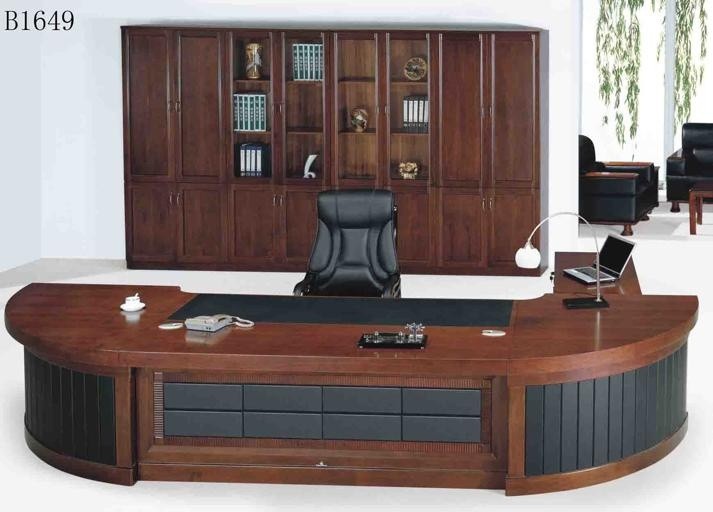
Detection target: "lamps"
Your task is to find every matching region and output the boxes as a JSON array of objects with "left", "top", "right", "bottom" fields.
[{"left": 515, "top": 212, "right": 610, "bottom": 310}]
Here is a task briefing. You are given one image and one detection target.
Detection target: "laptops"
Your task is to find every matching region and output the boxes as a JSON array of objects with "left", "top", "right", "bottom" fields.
[{"left": 563, "top": 233, "right": 636, "bottom": 284}]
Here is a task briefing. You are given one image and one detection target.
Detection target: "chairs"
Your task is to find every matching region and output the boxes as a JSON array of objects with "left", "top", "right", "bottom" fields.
[
  {"left": 294, "top": 190, "right": 400, "bottom": 298},
  {"left": 579, "top": 134, "right": 659, "bottom": 235},
  {"left": 665, "top": 123, "right": 713, "bottom": 212}
]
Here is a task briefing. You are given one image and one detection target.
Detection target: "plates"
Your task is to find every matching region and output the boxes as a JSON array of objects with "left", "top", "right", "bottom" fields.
[{"left": 120, "top": 302, "right": 146, "bottom": 312}]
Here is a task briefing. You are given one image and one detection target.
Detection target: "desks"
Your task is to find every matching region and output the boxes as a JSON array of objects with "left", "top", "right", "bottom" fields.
[
  {"left": 3, "top": 281, "right": 698, "bottom": 497},
  {"left": 554, "top": 251, "right": 642, "bottom": 296}
]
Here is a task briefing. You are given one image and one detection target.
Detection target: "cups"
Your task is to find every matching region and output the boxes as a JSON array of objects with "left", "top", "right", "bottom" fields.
[{"left": 126, "top": 296, "right": 140, "bottom": 309}]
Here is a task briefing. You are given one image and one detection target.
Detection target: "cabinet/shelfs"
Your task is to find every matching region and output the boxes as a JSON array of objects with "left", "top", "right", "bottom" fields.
[{"left": 119, "top": 25, "right": 548, "bottom": 278}]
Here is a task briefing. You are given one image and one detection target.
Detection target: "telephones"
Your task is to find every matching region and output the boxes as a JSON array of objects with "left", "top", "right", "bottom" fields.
[{"left": 185, "top": 313, "right": 255, "bottom": 333}]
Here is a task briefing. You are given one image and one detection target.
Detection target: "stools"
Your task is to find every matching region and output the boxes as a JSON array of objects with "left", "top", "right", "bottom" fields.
[{"left": 688, "top": 183, "right": 713, "bottom": 235}]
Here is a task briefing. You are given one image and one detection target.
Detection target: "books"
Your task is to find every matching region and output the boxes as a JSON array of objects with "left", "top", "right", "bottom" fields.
[
  {"left": 292, "top": 43, "right": 323, "bottom": 81},
  {"left": 234, "top": 94, "right": 267, "bottom": 132}
]
